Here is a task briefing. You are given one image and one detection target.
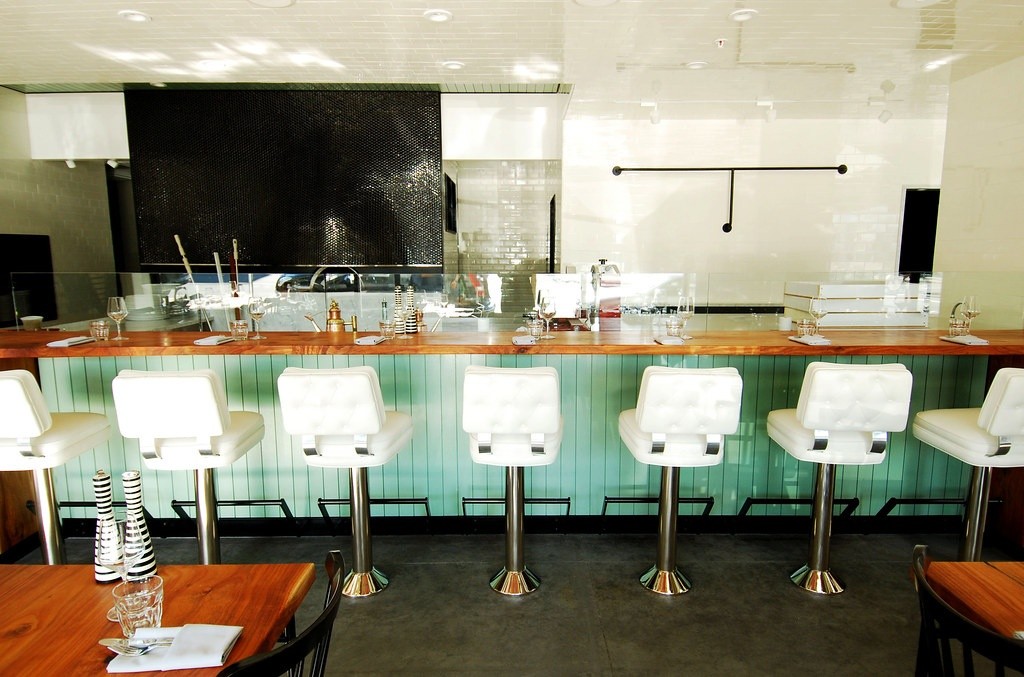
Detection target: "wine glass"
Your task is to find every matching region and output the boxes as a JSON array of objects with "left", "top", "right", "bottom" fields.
[
  {"left": 808, "top": 294, "right": 829, "bottom": 339},
  {"left": 960, "top": 295, "right": 982, "bottom": 337},
  {"left": 677, "top": 296, "right": 695, "bottom": 340},
  {"left": 539, "top": 296, "right": 557, "bottom": 340},
  {"left": 106, "top": 296, "right": 130, "bottom": 341},
  {"left": 97, "top": 511, "right": 146, "bottom": 623},
  {"left": 247, "top": 296, "right": 268, "bottom": 340},
  {"left": 174, "top": 286, "right": 191, "bottom": 324}
]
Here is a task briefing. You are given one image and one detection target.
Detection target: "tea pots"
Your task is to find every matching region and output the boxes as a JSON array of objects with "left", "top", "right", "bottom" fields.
[{"left": 303, "top": 298, "right": 358, "bottom": 333}]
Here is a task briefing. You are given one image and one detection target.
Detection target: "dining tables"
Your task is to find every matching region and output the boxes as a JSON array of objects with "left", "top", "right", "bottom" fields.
[
  {"left": 925, "top": 562, "right": 1024, "bottom": 677},
  {"left": 0, "top": 562, "right": 318, "bottom": 677}
]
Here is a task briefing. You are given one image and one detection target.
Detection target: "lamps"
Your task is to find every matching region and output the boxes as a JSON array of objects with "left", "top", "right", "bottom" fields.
[
  {"left": 765, "top": 104, "right": 777, "bottom": 123},
  {"left": 108, "top": 159, "right": 118, "bottom": 168},
  {"left": 880, "top": 110, "right": 892, "bottom": 124},
  {"left": 650, "top": 106, "right": 660, "bottom": 123},
  {"left": 66, "top": 160, "right": 76, "bottom": 168}
]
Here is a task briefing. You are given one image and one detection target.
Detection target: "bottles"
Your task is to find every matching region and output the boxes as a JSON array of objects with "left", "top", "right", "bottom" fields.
[
  {"left": 393, "top": 285, "right": 418, "bottom": 334},
  {"left": 93, "top": 469, "right": 123, "bottom": 584},
  {"left": 122, "top": 470, "right": 159, "bottom": 582}
]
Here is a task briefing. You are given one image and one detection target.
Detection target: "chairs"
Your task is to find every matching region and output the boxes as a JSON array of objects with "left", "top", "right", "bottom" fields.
[
  {"left": 910, "top": 544, "right": 1024, "bottom": 677},
  {"left": 215, "top": 550, "right": 346, "bottom": 677}
]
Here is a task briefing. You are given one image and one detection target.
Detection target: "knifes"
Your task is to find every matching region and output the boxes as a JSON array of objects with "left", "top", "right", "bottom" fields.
[{"left": 98, "top": 638, "right": 174, "bottom": 647}]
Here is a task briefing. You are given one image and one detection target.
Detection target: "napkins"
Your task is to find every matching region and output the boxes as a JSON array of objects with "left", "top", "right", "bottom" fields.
[{"left": 106, "top": 622, "right": 244, "bottom": 674}]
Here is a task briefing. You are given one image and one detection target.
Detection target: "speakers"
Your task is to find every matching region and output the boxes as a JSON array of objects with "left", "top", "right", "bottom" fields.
[{"left": 898, "top": 188, "right": 941, "bottom": 277}]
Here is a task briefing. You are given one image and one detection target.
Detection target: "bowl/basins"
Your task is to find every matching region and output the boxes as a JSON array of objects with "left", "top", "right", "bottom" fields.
[{"left": 20, "top": 315, "right": 44, "bottom": 331}]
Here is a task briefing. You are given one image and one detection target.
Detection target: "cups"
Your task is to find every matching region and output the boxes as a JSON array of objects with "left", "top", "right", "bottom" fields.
[
  {"left": 111, "top": 574, "right": 164, "bottom": 638},
  {"left": 89, "top": 316, "right": 972, "bottom": 342}
]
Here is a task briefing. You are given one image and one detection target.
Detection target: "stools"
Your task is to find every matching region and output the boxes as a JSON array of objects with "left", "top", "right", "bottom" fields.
[
  {"left": 461, "top": 365, "right": 560, "bottom": 596},
  {"left": 767, "top": 361, "right": 914, "bottom": 594},
  {"left": 911, "top": 365, "right": 1024, "bottom": 561},
  {"left": 113, "top": 369, "right": 265, "bottom": 565},
  {"left": 0, "top": 369, "right": 109, "bottom": 564},
  {"left": 277, "top": 366, "right": 411, "bottom": 597},
  {"left": 618, "top": 367, "right": 743, "bottom": 595}
]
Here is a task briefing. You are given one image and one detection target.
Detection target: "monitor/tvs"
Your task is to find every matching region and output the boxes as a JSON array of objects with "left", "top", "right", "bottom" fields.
[{"left": 0, "top": 235, "right": 60, "bottom": 328}]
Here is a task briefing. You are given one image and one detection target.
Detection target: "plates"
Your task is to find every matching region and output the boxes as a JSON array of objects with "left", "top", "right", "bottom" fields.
[{"left": 124, "top": 283, "right": 185, "bottom": 321}]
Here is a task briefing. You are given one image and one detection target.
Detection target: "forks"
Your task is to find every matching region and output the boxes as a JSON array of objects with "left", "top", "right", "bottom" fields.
[{"left": 107, "top": 641, "right": 172, "bottom": 656}]
